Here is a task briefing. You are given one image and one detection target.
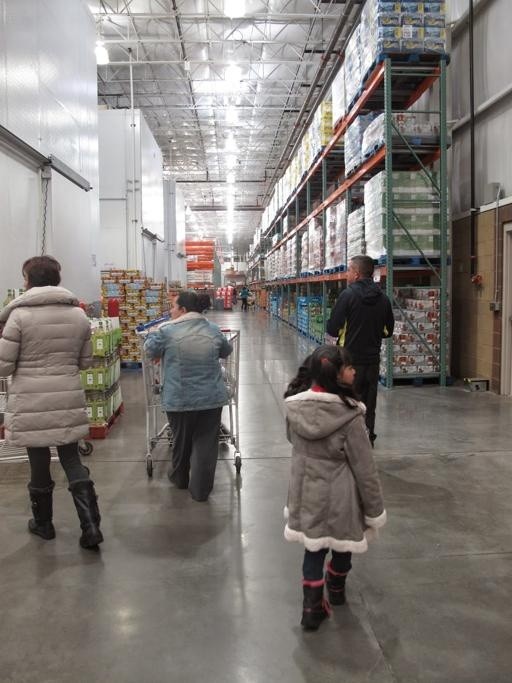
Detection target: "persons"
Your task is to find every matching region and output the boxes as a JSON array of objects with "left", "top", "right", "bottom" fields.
[
  {"left": 325, "top": 254, "right": 395, "bottom": 450},
  {"left": 281, "top": 345, "right": 387, "bottom": 629},
  {"left": 143, "top": 292, "right": 233, "bottom": 504},
  {"left": 232, "top": 286, "right": 249, "bottom": 310},
  {"left": 0, "top": 255, "right": 104, "bottom": 548}
]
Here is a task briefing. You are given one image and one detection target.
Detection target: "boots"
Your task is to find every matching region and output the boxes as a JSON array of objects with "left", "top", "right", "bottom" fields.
[
  {"left": 325, "top": 559, "right": 351, "bottom": 605},
  {"left": 68, "top": 478, "right": 103, "bottom": 548},
  {"left": 28, "top": 481, "right": 57, "bottom": 540},
  {"left": 300, "top": 579, "right": 332, "bottom": 628}
]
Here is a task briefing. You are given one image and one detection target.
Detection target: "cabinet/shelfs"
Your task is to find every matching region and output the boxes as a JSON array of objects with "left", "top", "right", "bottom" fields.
[{"left": 246, "top": 58, "right": 447, "bottom": 390}]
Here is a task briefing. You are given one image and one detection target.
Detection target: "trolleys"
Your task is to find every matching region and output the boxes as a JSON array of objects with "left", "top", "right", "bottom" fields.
[
  {"left": 142, "top": 325, "right": 242, "bottom": 480},
  {"left": 247, "top": 294, "right": 256, "bottom": 311},
  {"left": 0, "top": 372, "right": 94, "bottom": 463}
]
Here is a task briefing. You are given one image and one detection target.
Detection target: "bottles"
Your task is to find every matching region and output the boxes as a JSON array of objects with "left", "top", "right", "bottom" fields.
[
  {"left": 301, "top": 290, "right": 340, "bottom": 316},
  {"left": 79, "top": 316, "right": 122, "bottom": 420},
  {"left": 2, "top": 288, "right": 24, "bottom": 309}
]
